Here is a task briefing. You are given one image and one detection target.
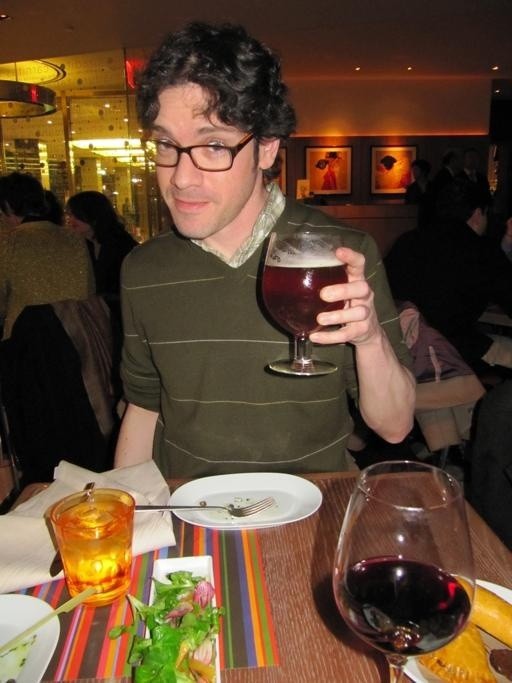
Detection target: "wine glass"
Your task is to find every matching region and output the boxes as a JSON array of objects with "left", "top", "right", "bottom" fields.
[
  {"left": 332, "top": 461, "right": 475, "bottom": 683},
  {"left": 262, "top": 233, "right": 348, "bottom": 377}
]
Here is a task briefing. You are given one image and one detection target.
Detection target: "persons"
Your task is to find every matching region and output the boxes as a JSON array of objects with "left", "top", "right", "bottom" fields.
[
  {"left": 113, "top": 24, "right": 416, "bottom": 488},
  {"left": 383, "top": 158, "right": 512, "bottom": 381},
  {"left": 2, "top": 173, "right": 138, "bottom": 446}
]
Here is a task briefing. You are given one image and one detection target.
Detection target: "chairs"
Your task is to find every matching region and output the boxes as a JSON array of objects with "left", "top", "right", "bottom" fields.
[{"left": 1, "top": 299, "right": 108, "bottom": 514}]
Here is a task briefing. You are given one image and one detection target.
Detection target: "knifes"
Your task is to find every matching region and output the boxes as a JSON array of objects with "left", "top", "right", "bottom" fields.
[{"left": 49, "top": 483, "right": 95, "bottom": 578}]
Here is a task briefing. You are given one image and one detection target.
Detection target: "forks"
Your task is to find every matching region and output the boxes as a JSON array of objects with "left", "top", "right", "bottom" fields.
[{"left": 135, "top": 497, "right": 273, "bottom": 516}]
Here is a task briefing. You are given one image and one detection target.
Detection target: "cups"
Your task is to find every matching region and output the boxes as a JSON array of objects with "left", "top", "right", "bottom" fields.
[{"left": 44, "top": 489, "right": 135, "bottom": 607}]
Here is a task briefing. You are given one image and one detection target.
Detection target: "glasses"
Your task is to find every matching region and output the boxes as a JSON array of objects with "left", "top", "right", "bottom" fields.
[{"left": 141, "top": 131, "right": 256, "bottom": 171}]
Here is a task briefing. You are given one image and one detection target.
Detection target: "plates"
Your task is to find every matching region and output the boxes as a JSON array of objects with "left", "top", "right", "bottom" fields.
[
  {"left": 402, "top": 574, "right": 512, "bottom": 683},
  {"left": 0, "top": 594, "right": 60, "bottom": 683},
  {"left": 170, "top": 473, "right": 324, "bottom": 529}
]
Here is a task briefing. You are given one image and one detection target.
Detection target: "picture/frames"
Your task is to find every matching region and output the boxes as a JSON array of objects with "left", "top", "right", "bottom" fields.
[
  {"left": 305, "top": 145, "right": 353, "bottom": 197},
  {"left": 263, "top": 146, "right": 288, "bottom": 196},
  {"left": 369, "top": 144, "right": 418, "bottom": 196}
]
